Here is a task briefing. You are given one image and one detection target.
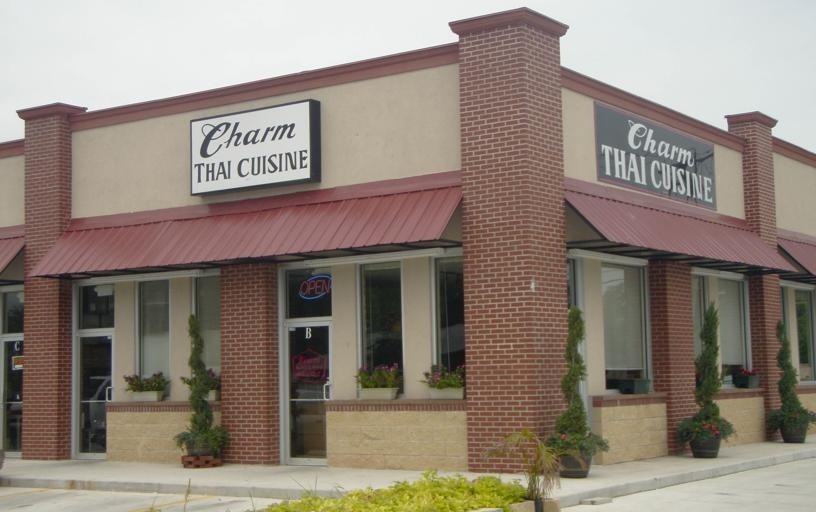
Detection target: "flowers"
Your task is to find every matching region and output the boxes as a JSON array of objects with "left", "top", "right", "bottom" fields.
[
  {"left": 417, "top": 364, "right": 466, "bottom": 389},
  {"left": 354, "top": 364, "right": 401, "bottom": 388},
  {"left": 740, "top": 368, "right": 758, "bottom": 376},
  {"left": 542, "top": 304, "right": 608, "bottom": 453},
  {"left": 678, "top": 301, "right": 737, "bottom": 443}
]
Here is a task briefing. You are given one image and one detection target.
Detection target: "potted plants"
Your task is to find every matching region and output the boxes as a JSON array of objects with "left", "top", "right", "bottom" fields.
[
  {"left": 122, "top": 314, "right": 230, "bottom": 459},
  {"left": 769, "top": 320, "right": 816, "bottom": 443}
]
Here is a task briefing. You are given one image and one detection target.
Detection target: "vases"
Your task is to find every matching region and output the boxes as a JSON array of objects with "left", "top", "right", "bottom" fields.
[
  {"left": 690, "top": 439, "right": 721, "bottom": 458},
  {"left": 429, "top": 389, "right": 465, "bottom": 400},
  {"left": 560, "top": 453, "right": 591, "bottom": 477},
  {"left": 360, "top": 388, "right": 397, "bottom": 399},
  {"left": 739, "top": 376, "right": 759, "bottom": 388},
  {"left": 619, "top": 378, "right": 651, "bottom": 394}
]
